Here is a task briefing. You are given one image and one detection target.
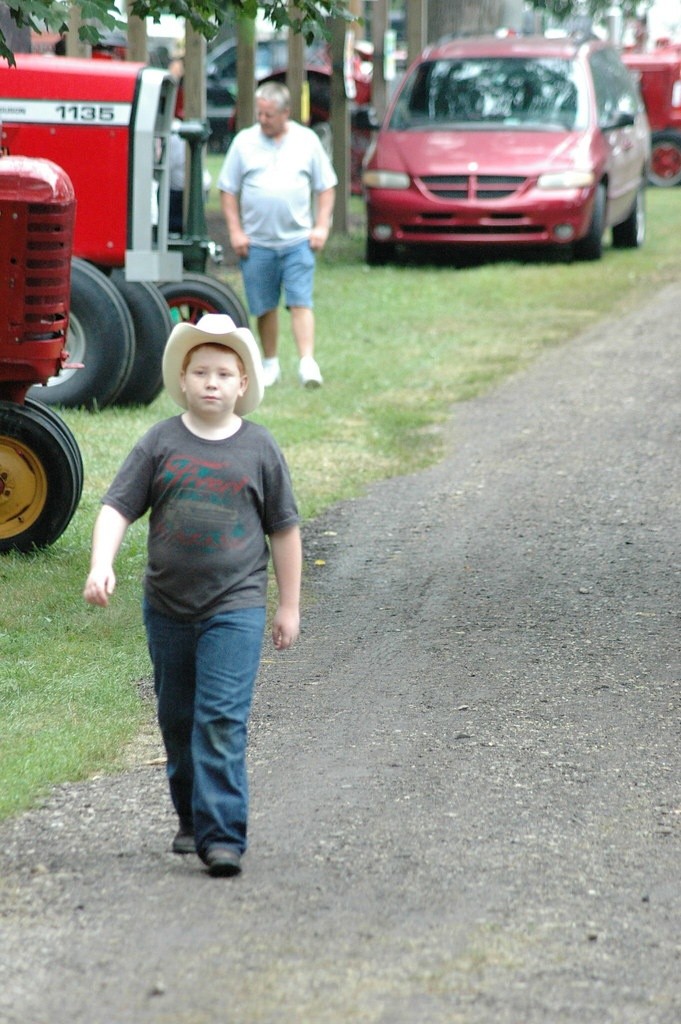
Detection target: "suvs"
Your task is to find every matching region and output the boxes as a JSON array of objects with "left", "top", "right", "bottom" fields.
[{"left": 351, "top": 26, "right": 650, "bottom": 269}]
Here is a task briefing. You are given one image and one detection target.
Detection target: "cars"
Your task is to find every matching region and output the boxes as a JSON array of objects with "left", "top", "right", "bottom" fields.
[{"left": 95, "top": 21, "right": 681, "bottom": 186}]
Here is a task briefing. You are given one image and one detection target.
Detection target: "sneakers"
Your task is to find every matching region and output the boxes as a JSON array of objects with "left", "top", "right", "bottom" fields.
[
  {"left": 299, "top": 357, "right": 322, "bottom": 388},
  {"left": 262, "top": 359, "right": 280, "bottom": 385}
]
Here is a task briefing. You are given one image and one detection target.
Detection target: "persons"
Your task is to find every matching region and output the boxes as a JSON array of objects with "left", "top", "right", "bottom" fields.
[
  {"left": 82, "top": 313, "right": 303, "bottom": 878},
  {"left": 214, "top": 82, "right": 338, "bottom": 390}
]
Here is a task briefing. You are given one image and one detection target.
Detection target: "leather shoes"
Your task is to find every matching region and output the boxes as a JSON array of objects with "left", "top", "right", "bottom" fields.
[
  {"left": 173, "top": 828, "right": 198, "bottom": 853},
  {"left": 207, "top": 848, "right": 243, "bottom": 876}
]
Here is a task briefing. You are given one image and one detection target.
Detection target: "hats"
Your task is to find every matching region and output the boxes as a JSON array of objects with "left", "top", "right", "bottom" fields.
[{"left": 161, "top": 313, "right": 263, "bottom": 416}]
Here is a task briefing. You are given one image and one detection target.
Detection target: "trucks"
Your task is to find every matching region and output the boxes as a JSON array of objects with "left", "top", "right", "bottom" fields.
[
  {"left": 0, "top": 52, "right": 183, "bottom": 410},
  {"left": 0, "top": 123, "right": 85, "bottom": 560},
  {"left": 152, "top": 115, "right": 251, "bottom": 332}
]
